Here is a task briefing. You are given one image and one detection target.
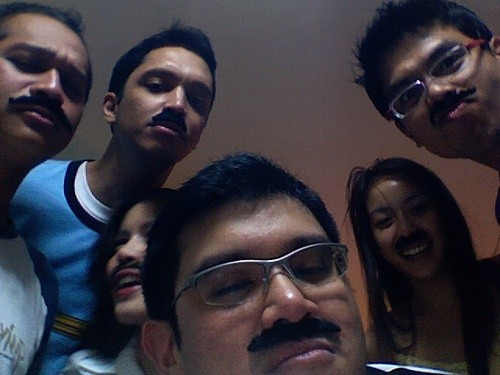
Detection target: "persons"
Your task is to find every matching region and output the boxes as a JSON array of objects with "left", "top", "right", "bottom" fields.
[
  {"left": 140, "top": 152, "right": 452, "bottom": 374},
  {"left": 0, "top": 1, "right": 217, "bottom": 374},
  {"left": 346, "top": 0, "right": 500, "bottom": 375}
]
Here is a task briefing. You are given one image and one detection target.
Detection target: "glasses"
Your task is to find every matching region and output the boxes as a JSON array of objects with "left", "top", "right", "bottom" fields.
[
  {"left": 171, "top": 242, "right": 349, "bottom": 308},
  {"left": 384, "top": 38, "right": 485, "bottom": 122}
]
